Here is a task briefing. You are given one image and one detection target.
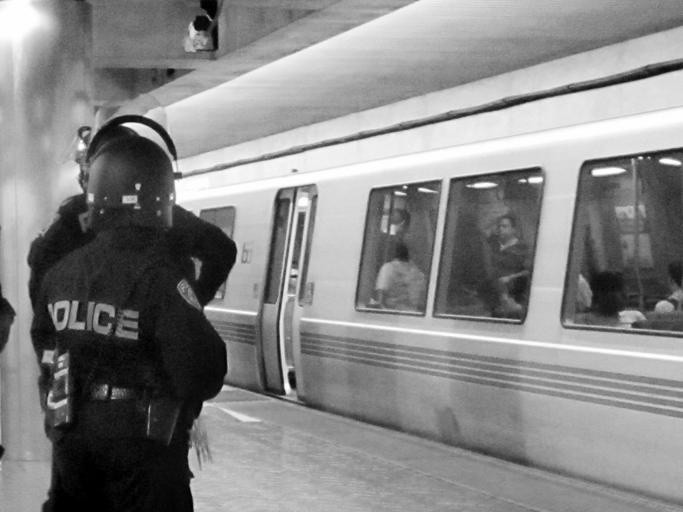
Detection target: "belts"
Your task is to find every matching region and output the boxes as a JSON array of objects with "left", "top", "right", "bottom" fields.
[{"left": 86, "top": 382, "right": 152, "bottom": 401}]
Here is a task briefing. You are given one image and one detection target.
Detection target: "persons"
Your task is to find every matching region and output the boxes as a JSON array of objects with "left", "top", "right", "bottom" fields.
[
  {"left": 574, "top": 270, "right": 647, "bottom": 326},
  {"left": 368, "top": 236, "right": 423, "bottom": 309},
  {"left": 495, "top": 268, "right": 530, "bottom": 317},
  {"left": 488, "top": 216, "right": 527, "bottom": 268},
  {"left": 31, "top": 134, "right": 228, "bottom": 512},
  {"left": 28, "top": 128, "right": 237, "bottom": 361},
  {"left": 655, "top": 257, "right": 683, "bottom": 314}
]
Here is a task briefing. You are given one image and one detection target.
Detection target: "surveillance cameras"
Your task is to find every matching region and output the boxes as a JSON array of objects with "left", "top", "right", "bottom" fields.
[{"left": 187, "top": 13, "right": 215, "bottom": 50}]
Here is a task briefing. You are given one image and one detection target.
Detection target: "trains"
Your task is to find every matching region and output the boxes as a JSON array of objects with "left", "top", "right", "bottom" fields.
[{"left": 169, "top": 70, "right": 683, "bottom": 508}]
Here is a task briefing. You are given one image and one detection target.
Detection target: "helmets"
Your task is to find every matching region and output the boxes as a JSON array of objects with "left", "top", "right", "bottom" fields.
[{"left": 75, "top": 126, "right": 175, "bottom": 228}]
